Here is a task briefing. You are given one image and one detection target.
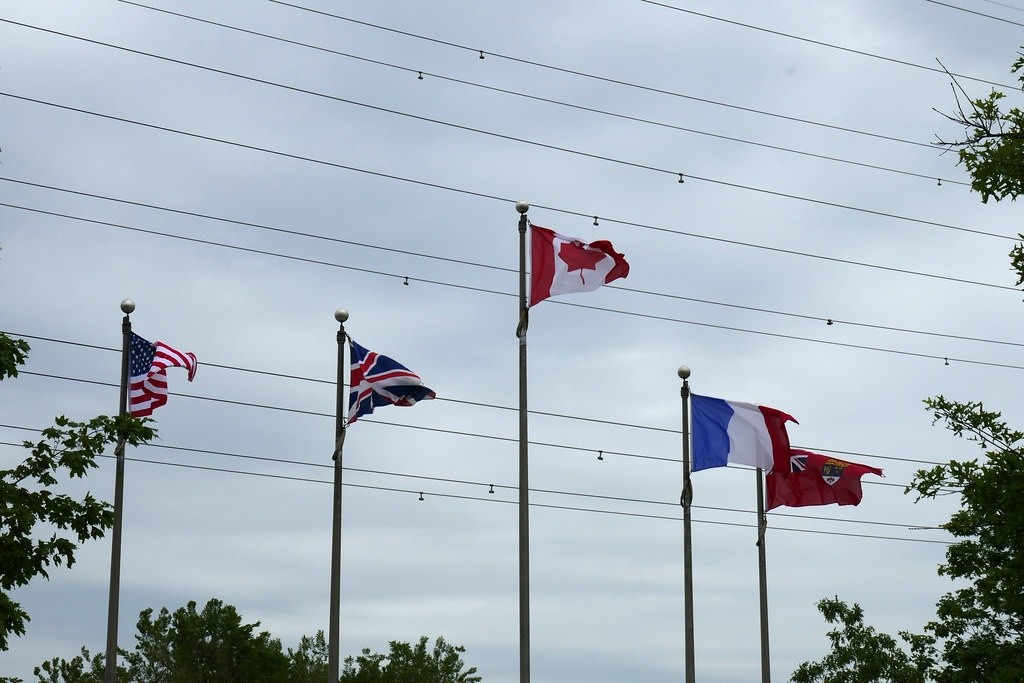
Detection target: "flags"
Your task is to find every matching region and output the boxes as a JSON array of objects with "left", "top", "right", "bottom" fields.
[
  {"left": 690, "top": 393, "right": 800, "bottom": 472},
  {"left": 765, "top": 449, "right": 887, "bottom": 512},
  {"left": 527, "top": 220, "right": 630, "bottom": 307},
  {"left": 127, "top": 330, "right": 197, "bottom": 416},
  {"left": 343, "top": 331, "right": 436, "bottom": 429}
]
